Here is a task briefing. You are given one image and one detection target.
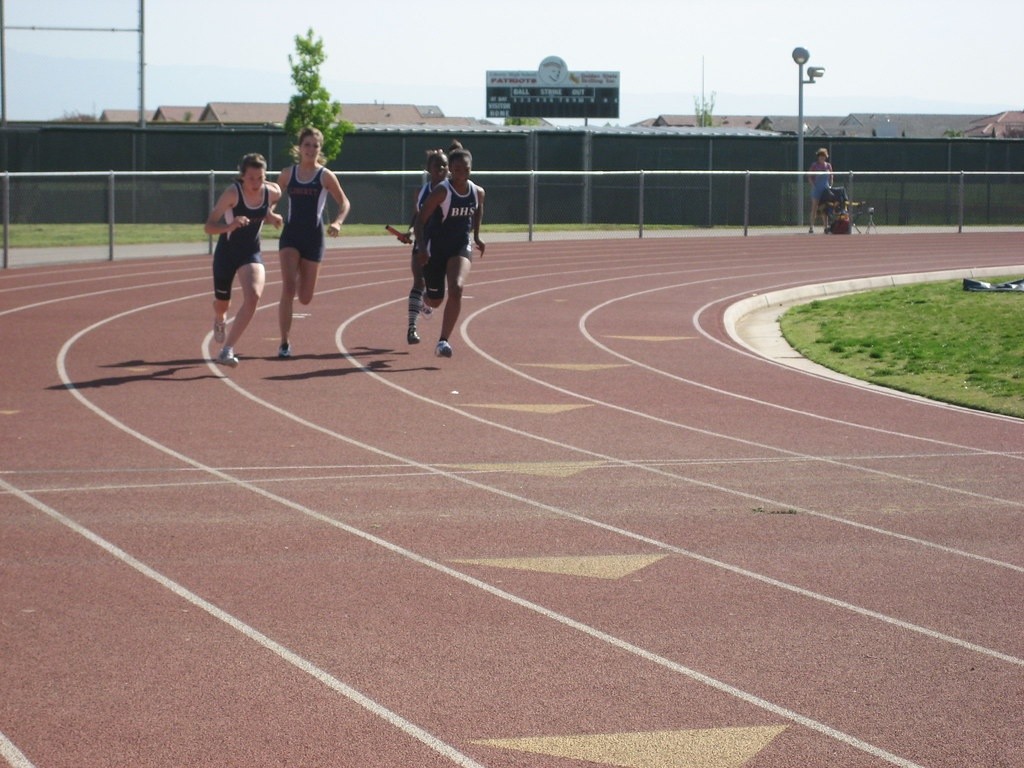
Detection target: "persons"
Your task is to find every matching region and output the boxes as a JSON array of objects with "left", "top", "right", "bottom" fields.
[
  {"left": 265, "top": 126, "right": 351, "bottom": 360},
  {"left": 203, "top": 152, "right": 285, "bottom": 368},
  {"left": 395, "top": 140, "right": 487, "bottom": 358},
  {"left": 832, "top": 201, "right": 844, "bottom": 216},
  {"left": 807, "top": 148, "right": 834, "bottom": 234}
]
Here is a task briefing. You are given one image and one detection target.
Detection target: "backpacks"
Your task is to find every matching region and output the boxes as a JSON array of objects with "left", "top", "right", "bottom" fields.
[{"left": 819, "top": 186, "right": 851, "bottom": 235}]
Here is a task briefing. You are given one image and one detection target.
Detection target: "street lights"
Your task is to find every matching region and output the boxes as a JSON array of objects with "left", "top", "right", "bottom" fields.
[{"left": 792, "top": 47, "right": 825, "bottom": 225}]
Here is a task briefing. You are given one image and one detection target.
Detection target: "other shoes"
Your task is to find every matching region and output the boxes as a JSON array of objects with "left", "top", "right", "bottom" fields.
[
  {"left": 407, "top": 330, "right": 420, "bottom": 344},
  {"left": 823, "top": 229, "right": 829, "bottom": 235},
  {"left": 808, "top": 227, "right": 813, "bottom": 233},
  {"left": 422, "top": 303, "right": 433, "bottom": 316}
]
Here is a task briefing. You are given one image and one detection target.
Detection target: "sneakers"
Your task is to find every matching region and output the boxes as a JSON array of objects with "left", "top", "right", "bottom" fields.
[
  {"left": 217, "top": 345, "right": 239, "bottom": 367},
  {"left": 278, "top": 344, "right": 291, "bottom": 360},
  {"left": 435, "top": 340, "right": 452, "bottom": 357},
  {"left": 213, "top": 320, "right": 226, "bottom": 343}
]
